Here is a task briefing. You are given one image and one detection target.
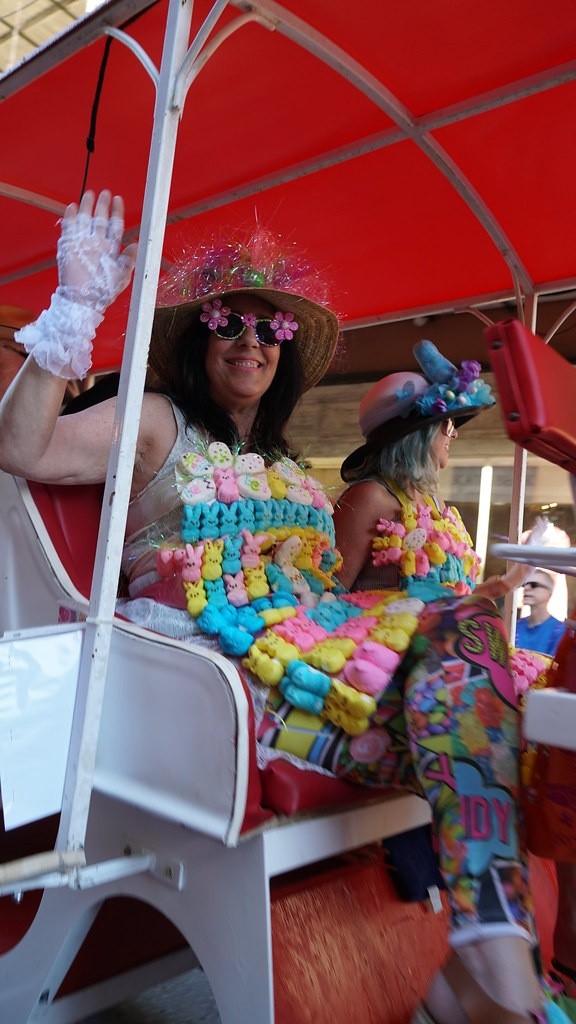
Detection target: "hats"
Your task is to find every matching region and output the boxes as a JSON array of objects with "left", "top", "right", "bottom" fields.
[
  {"left": 339, "top": 339, "right": 497, "bottom": 483},
  {"left": 121, "top": 206, "right": 350, "bottom": 396}
]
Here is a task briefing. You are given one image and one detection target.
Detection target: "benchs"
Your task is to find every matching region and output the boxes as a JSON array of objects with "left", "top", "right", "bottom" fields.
[{"left": 0, "top": 473, "right": 432, "bottom": 1024}]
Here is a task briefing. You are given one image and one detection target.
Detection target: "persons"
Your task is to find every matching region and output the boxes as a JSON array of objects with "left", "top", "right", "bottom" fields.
[
  {"left": 0, "top": 190, "right": 576, "bottom": 1024},
  {"left": 516, "top": 570, "right": 566, "bottom": 655},
  {"left": 332, "top": 373, "right": 553, "bottom": 599}
]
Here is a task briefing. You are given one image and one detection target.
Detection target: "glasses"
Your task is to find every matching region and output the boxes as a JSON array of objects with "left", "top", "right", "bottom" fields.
[
  {"left": 443, "top": 416, "right": 455, "bottom": 437},
  {"left": 522, "top": 580, "right": 547, "bottom": 590},
  {"left": 214, "top": 309, "right": 285, "bottom": 346}
]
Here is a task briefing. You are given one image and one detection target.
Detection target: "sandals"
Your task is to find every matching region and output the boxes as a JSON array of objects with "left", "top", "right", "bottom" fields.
[{"left": 412, "top": 922, "right": 548, "bottom": 1024}]
